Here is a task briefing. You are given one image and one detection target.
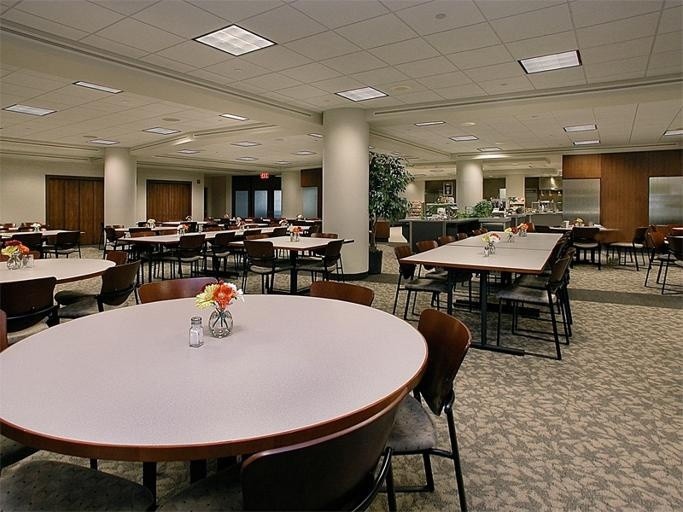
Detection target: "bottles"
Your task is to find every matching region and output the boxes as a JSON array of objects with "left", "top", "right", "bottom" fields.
[{"left": 189, "top": 317, "right": 205, "bottom": 347}]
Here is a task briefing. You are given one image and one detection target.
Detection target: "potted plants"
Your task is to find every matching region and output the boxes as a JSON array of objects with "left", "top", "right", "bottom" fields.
[{"left": 369, "top": 150, "right": 416, "bottom": 275}]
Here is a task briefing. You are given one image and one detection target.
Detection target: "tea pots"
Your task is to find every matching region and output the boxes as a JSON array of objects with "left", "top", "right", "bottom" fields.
[{"left": 177, "top": 228, "right": 185, "bottom": 234}]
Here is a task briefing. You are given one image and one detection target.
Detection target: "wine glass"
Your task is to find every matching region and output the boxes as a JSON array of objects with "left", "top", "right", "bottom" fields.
[{"left": 483, "top": 243, "right": 489, "bottom": 257}]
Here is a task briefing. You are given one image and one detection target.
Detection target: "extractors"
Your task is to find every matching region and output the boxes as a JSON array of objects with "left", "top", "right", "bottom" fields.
[{"left": 537, "top": 178, "right": 563, "bottom": 192}]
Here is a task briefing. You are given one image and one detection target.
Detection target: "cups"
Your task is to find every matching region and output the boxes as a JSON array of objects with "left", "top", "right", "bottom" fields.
[
  {"left": 560, "top": 220, "right": 569, "bottom": 227},
  {"left": 22, "top": 255, "right": 34, "bottom": 269}
]
